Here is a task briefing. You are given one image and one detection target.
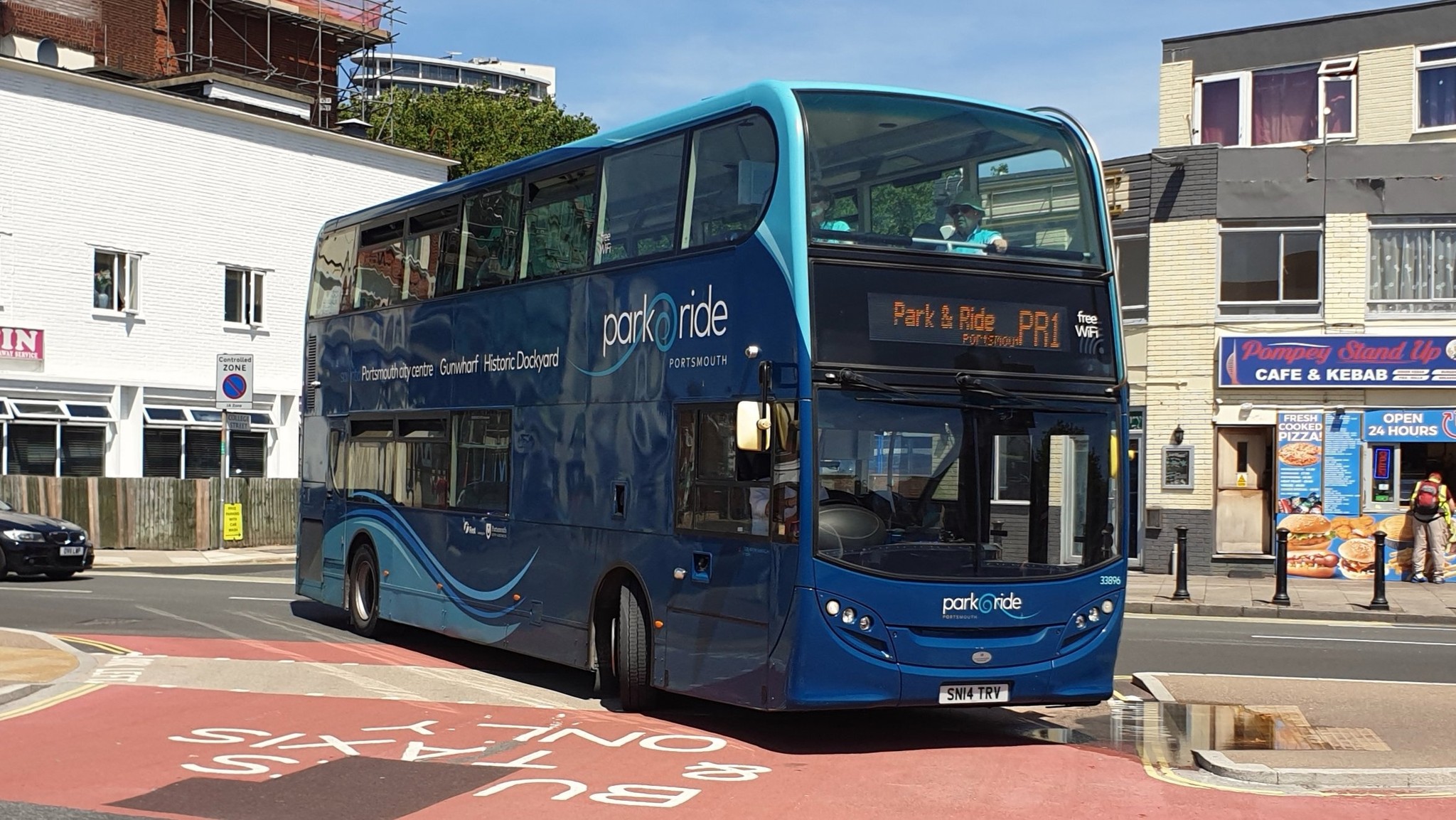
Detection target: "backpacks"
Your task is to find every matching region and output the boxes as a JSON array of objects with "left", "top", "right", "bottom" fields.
[{"left": 1413, "top": 478, "right": 1442, "bottom": 515}]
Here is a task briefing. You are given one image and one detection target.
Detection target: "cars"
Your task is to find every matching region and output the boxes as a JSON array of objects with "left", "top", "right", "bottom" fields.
[{"left": 0, "top": 500, "right": 95, "bottom": 582}]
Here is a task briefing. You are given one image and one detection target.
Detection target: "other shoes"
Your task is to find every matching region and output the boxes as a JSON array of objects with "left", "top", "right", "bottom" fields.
[
  {"left": 1411, "top": 575, "right": 1428, "bottom": 583},
  {"left": 1435, "top": 578, "right": 1445, "bottom": 584}
]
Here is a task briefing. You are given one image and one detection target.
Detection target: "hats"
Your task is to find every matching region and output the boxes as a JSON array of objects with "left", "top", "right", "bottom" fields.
[{"left": 949, "top": 190, "right": 986, "bottom": 218}]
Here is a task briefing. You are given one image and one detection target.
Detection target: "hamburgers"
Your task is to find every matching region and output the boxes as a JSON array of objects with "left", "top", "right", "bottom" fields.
[
  {"left": 1277, "top": 514, "right": 1336, "bottom": 551},
  {"left": 1337, "top": 538, "right": 1376, "bottom": 580}
]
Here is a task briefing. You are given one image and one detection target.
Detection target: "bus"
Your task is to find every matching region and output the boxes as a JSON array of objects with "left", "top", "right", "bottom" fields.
[{"left": 289, "top": 78, "right": 1131, "bottom": 722}]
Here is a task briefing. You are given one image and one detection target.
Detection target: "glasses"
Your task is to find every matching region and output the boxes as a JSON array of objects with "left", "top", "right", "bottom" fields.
[
  {"left": 810, "top": 197, "right": 823, "bottom": 203},
  {"left": 950, "top": 206, "right": 972, "bottom": 214}
]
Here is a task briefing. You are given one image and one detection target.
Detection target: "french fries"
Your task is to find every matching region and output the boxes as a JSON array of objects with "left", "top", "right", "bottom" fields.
[{"left": 1387, "top": 547, "right": 1456, "bottom": 580}]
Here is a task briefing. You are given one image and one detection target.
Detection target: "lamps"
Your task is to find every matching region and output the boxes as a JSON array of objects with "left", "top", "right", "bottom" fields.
[{"left": 1174, "top": 425, "right": 1184, "bottom": 445}]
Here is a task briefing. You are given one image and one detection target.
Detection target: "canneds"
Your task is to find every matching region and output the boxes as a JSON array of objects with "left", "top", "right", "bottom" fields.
[{"left": 1278, "top": 492, "right": 1322, "bottom": 514}]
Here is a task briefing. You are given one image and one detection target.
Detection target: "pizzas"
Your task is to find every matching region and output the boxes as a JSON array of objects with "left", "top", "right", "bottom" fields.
[{"left": 1278, "top": 443, "right": 1320, "bottom": 465}]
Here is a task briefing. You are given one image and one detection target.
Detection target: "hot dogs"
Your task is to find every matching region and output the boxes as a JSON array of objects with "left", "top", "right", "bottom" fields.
[{"left": 1287, "top": 550, "right": 1339, "bottom": 578}]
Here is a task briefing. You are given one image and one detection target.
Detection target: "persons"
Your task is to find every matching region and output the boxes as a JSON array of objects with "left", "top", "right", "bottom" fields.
[
  {"left": 1410, "top": 472, "right": 1456, "bottom": 585},
  {"left": 807, "top": 184, "right": 853, "bottom": 245},
  {"left": 750, "top": 453, "right": 829, "bottom": 535},
  {"left": 934, "top": 190, "right": 1008, "bottom": 256}
]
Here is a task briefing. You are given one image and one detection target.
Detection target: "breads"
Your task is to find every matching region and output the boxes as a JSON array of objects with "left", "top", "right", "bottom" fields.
[{"left": 1377, "top": 515, "right": 1414, "bottom": 541}]
